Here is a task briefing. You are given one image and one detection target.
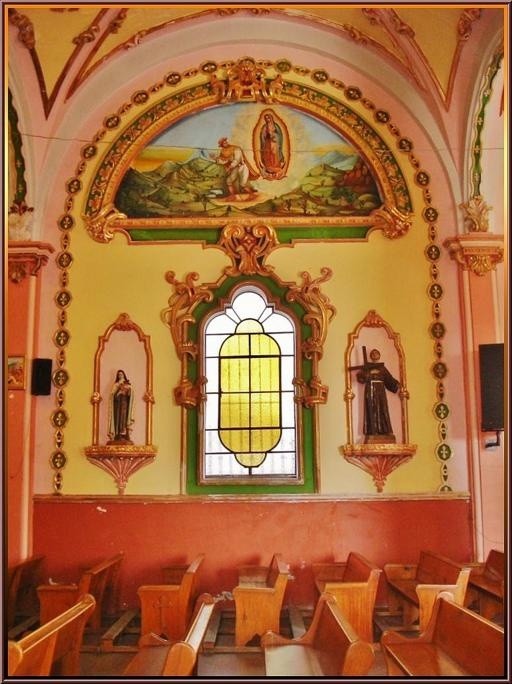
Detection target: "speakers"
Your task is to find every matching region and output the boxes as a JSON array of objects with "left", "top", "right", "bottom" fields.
[
  {"left": 30, "top": 358, "right": 52, "bottom": 395},
  {"left": 478, "top": 342, "right": 504, "bottom": 435}
]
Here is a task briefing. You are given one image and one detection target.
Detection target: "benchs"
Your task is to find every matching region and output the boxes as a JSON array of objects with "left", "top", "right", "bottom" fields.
[
  {"left": 461, "top": 550, "right": 509, "bottom": 623},
  {"left": 223, "top": 552, "right": 293, "bottom": 650},
  {"left": 4, "top": 593, "right": 97, "bottom": 677},
  {"left": 311, "top": 549, "right": 383, "bottom": 646},
  {"left": 252, "top": 592, "right": 377, "bottom": 678},
  {"left": 384, "top": 586, "right": 508, "bottom": 678},
  {"left": 382, "top": 551, "right": 474, "bottom": 634},
  {"left": 133, "top": 551, "right": 207, "bottom": 646},
  {"left": 5, "top": 549, "right": 48, "bottom": 642},
  {"left": 36, "top": 551, "right": 121, "bottom": 637},
  {"left": 115, "top": 593, "right": 220, "bottom": 678}
]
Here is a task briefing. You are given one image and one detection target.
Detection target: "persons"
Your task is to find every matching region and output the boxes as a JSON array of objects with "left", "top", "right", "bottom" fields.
[
  {"left": 111, "top": 370, "right": 134, "bottom": 439},
  {"left": 260, "top": 113, "right": 283, "bottom": 172},
  {"left": 355, "top": 349, "right": 401, "bottom": 434},
  {"left": 217, "top": 137, "right": 250, "bottom": 195}
]
